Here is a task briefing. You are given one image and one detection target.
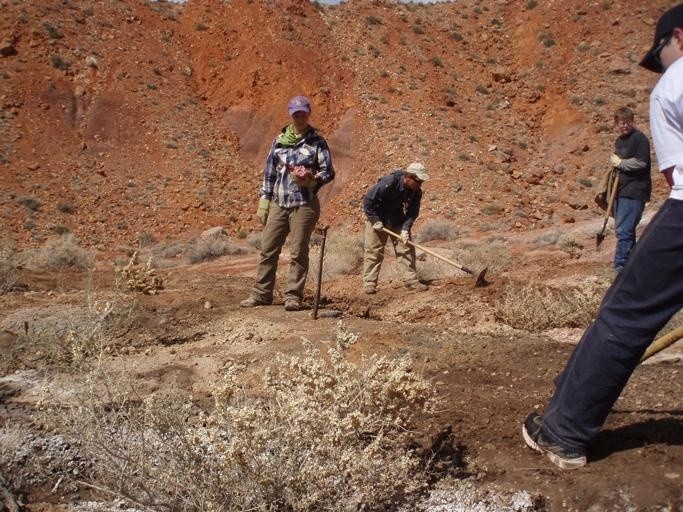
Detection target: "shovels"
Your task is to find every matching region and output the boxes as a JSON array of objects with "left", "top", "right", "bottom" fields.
[{"left": 597, "top": 171, "right": 621, "bottom": 251}]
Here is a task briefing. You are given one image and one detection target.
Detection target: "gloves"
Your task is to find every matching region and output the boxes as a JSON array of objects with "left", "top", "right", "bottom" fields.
[
  {"left": 373, "top": 221, "right": 383, "bottom": 232},
  {"left": 255, "top": 198, "right": 270, "bottom": 225},
  {"left": 288, "top": 173, "right": 318, "bottom": 189},
  {"left": 400, "top": 229, "right": 411, "bottom": 246},
  {"left": 610, "top": 153, "right": 622, "bottom": 168}
]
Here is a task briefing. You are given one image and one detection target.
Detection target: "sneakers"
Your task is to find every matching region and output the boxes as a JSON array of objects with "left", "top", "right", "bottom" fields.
[
  {"left": 239, "top": 297, "right": 273, "bottom": 307},
  {"left": 403, "top": 280, "right": 429, "bottom": 291},
  {"left": 522, "top": 411, "right": 590, "bottom": 470},
  {"left": 284, "top": 299, "right": 300, "bottom": 311},
  {"left": 364, "top": 285, "right": 377, "bottom": 294}
]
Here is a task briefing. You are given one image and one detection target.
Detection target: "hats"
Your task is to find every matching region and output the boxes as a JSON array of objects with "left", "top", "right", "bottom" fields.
[
  {"left": 288, "top": 95, "right": 312, "bottom": 118},
  {"left": 638, "top": 5, "right": 683, "bottom": 75},
  {"left": 408, "top": 162, "right": 430, "bottom": 182}
]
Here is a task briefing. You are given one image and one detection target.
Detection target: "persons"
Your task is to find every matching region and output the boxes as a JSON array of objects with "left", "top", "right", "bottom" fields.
[
  {"left": 610, "top": 107, "right": 652, "bottom": 274},
  {"left": 521, "top": 6, "right": 683, "bottom": 472},
  {"left": 240, "top": 95, "right": 335, "bottom": 312},
  {"left": 362, "top": 162, "right": 429, "bottom": 293}
]
